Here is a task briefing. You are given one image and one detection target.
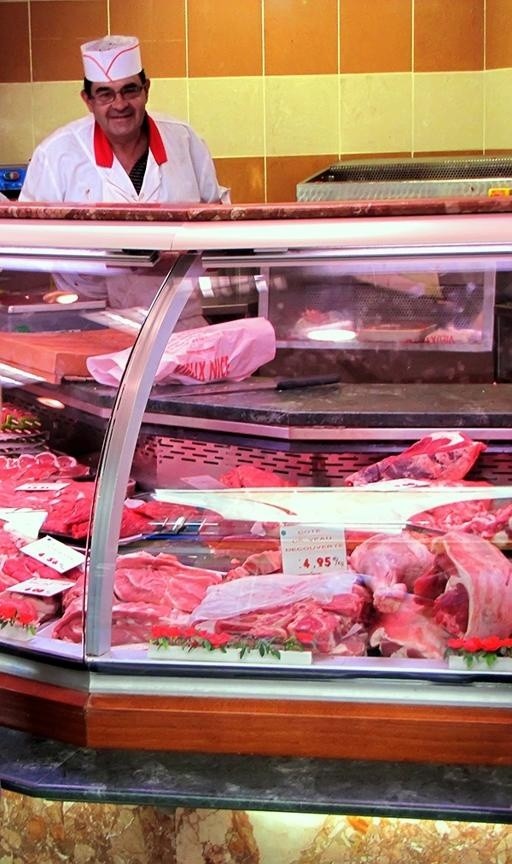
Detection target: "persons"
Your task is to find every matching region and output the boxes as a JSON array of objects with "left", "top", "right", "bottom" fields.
[{"left": 17, "top": 36, "right": 225, "bottom": 333}]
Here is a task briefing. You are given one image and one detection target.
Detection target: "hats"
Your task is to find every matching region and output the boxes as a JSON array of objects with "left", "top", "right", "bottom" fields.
[{"left": 79, "top": 34, "right": 144, "bottom": 83}]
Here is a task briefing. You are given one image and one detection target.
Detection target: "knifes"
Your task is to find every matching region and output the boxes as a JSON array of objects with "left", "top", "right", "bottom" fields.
[{"left": 170, "top": 369, "right": 339, "bottom": 396}]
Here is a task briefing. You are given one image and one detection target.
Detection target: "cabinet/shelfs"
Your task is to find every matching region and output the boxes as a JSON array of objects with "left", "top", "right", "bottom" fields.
[{"left": 0, "top": 194, "right": 512, "bottom": 825}]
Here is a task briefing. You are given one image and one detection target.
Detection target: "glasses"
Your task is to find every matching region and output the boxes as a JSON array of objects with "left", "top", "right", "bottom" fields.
[{"left": 87, "top": 80, "right": 147, "bottom": 105}]
